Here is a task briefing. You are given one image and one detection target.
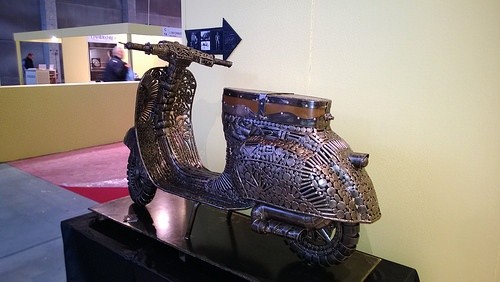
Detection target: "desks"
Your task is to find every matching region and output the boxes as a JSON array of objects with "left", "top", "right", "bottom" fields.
[
  {"left": 24, "top": 68, "right": 56, "bottom": 85},
  {"left": 60, "top": 188, "right": 420, "bottom": 282}
]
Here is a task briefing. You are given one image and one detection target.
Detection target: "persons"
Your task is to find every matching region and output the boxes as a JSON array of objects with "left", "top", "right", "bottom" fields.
[
  {"left": 25, "top": 52, "right": 36, "bottom": 69},
  {"left": 101, "top": 46, "right": 131, "bottom": 82}
]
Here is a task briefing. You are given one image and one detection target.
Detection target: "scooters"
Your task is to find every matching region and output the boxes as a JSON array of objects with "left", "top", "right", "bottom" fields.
[{"left": 118, "top": 40, "right": 382, "bottom": 273}]
